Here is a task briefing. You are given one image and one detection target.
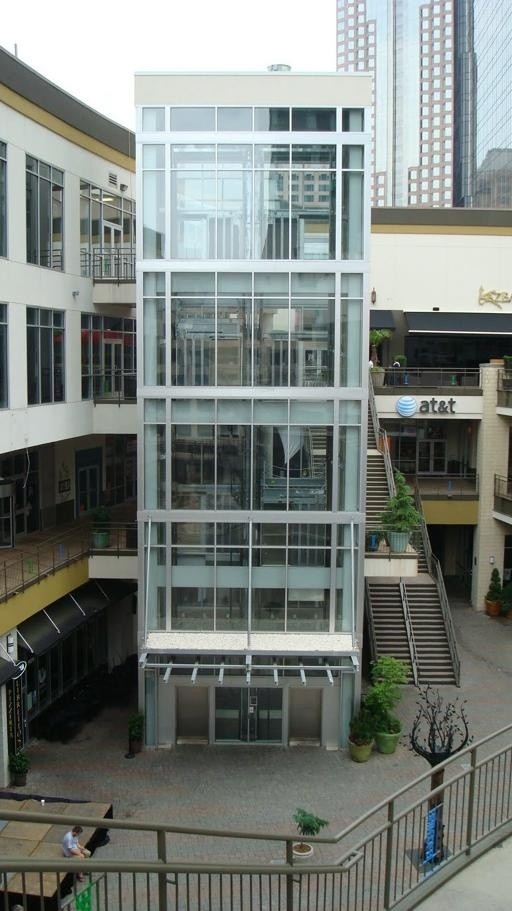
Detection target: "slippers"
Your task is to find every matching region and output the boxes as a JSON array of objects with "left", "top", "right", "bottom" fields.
[{"left": 77, "top": 872, "right": 90, "bottom": 882}]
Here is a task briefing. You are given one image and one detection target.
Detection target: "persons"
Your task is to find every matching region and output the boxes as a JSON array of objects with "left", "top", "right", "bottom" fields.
[
  {"left": 368, "top": 358, "right": 410, "bottom": 386},
  {"left": 62, "top": 826, "right": 91, "bottom": 882}
]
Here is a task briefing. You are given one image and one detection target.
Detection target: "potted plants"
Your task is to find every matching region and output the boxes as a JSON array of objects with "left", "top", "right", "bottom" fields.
[
  {"left": 485, "top": 566, "right": 504, "bottom": 616},
  {"left": 381, "top": 464, "right": 421, "bottom": 553},
  {"left": 292, "top": 806, "right": 328, "bottom": 860},
  {"left": 394, "top": 354, "right": 407, "bottom": 384},
  {"left": 367, "top": 655, "right": 411, "bottom": 754},
  {"left": 503, "top": 567, "right": 512, "bottom": 619},
  {"left": 348, "top": 715, "right": 375, "bottom": 762},
  {"left": 128, "top": 711, "right": 145, "bottom": 753},
  {"left": 8, "top": 750, "right": 32, "bottom": 787},
  {"left": 91, "top": 504, "right": 111, "bottom": 547},
  {"left": 370, "top": 327, "right": 391, "bottom": 386}
]
